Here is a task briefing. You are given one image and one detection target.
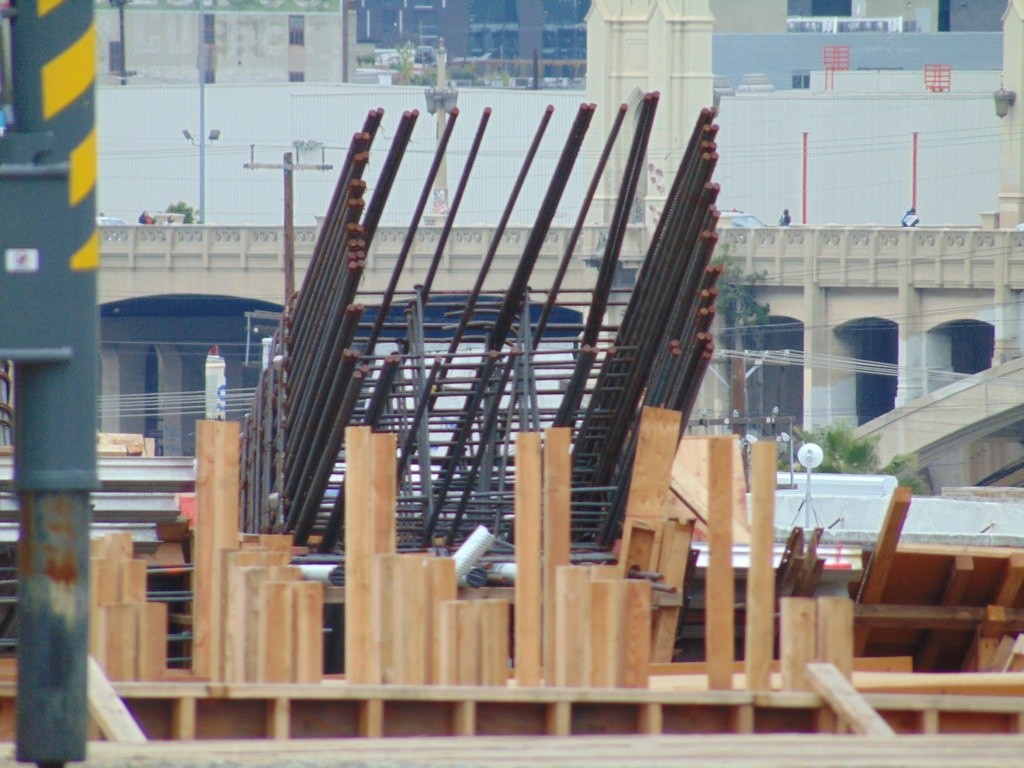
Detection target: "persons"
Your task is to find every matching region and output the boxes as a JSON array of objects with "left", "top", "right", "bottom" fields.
[
  {"left": 778, "top": 209, "right": 791, "bottom": 227},
  {"left": 138, "top": 210, "right": 155, "bottom": 225}
]
